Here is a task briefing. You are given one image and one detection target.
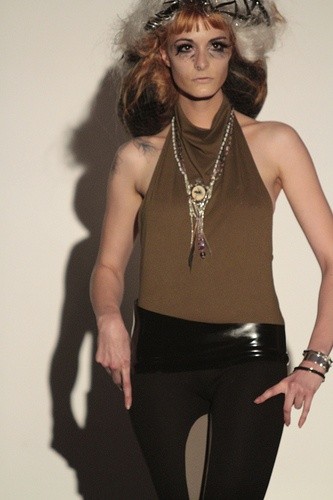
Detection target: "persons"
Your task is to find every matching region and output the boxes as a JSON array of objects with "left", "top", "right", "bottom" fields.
[{"left": 89, "top": 0, "right": 333, "bottom": 500}]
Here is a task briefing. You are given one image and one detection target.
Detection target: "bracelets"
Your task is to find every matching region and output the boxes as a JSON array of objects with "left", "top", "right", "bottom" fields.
[
  {"left": 302, "top": 349, "right": 333, "bottom": 373},
  {"left": 294, "top": 366, "right": 326, "bottom": 385}
]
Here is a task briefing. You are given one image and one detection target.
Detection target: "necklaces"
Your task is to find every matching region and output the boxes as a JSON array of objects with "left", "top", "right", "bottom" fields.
[{"left": 171, "top": 110, "right": 236, "bottom": 270}]
